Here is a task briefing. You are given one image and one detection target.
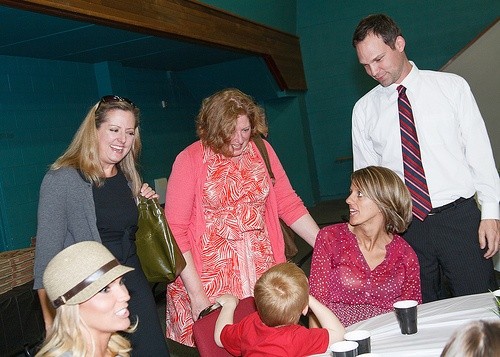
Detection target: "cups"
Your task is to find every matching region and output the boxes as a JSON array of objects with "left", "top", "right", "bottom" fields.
[
  {"left": 329, "top": 340, "right": 359, "bottom": 357},
  {"left": 493, "top": 290, "right": 500, "bottom": 312},
  {"left": 343, "top": 330, "right": 371, "bottom": 355},
  {"left": 393, "top": 300, "right": 418, "bottom": 335}
]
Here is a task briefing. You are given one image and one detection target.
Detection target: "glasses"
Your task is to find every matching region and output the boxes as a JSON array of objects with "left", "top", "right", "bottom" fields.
[{"left": 96, "top": 95, "right": 137, "bottom": 116}]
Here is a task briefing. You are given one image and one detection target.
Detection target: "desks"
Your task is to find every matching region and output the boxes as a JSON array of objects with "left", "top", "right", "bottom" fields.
[
  {"left": 309, "top": 290, "right": 500, "bottom": 357},
  {"left": 0, "top": 243, "right": 46, "bottom": 357}
]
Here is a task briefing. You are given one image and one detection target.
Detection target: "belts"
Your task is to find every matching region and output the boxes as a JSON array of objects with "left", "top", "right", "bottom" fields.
[{"left": 429, "top": 196, "right": 472, "bottom": 216}]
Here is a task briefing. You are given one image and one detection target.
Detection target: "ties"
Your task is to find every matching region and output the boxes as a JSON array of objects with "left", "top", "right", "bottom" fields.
[{"left": 396, "top": 84, "right": 433, "bottom": 222}]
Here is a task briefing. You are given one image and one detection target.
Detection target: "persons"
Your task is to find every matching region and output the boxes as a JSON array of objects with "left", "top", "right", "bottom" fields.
[
  {"left": 166, "top": 89, "right": 320, "bottom": 347},
  {"left": 352, "top": 15, "right": 500, "bottom": 302},
  {"left": 33, "top": 95, "right": 169, "bottom": 357},
  {"left": 214, "top": 262, "right": 345, "bottom": 357},
  {"left": 34, "top": 241, "right": 135, "bottom": 357},
  {"left": 309, "top": 167, "right": 423, "bottom": 328},
  {"left": 440, "top": 319, "right": 500, "bottom": 357}
]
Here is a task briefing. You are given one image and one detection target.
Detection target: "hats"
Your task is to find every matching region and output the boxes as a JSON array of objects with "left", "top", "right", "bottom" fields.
[{"left": 42, "top": 241, "right": 136, "bottom": 309}]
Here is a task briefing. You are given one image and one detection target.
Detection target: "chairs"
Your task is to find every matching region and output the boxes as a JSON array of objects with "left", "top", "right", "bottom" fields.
[{"left": 190, "top": 296, "right": 255, "bottom": 357}]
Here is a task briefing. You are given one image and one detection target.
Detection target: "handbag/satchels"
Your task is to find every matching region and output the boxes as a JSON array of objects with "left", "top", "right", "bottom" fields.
[
  {"left": 135, "top": 192, "right": 187, "bottom": 283},
  {"left": 276, "top": 218, "right": 299, "bottom": 259}
]
